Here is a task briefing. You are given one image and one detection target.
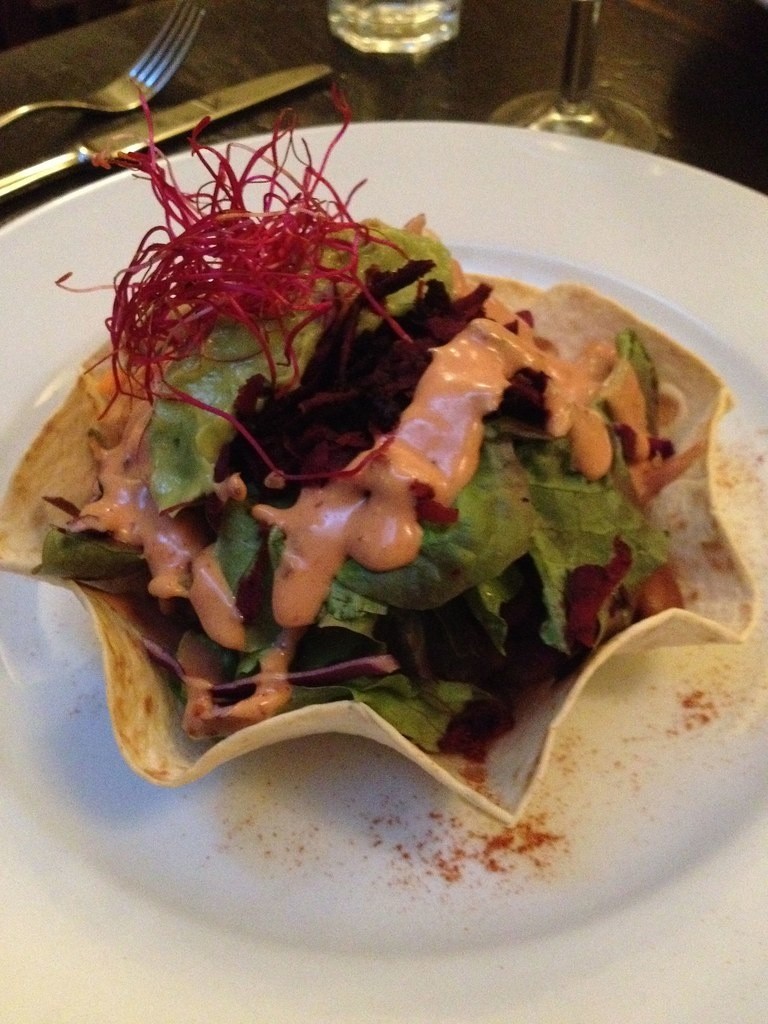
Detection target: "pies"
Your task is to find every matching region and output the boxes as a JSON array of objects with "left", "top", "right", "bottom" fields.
[{"left": 1, "top": 266, "right": 760, "bottom": 830}]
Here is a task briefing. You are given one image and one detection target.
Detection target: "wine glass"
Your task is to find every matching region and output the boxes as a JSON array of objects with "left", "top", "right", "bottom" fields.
[{"left": 489, "top": 0, "right": 658, "bottom": 155}]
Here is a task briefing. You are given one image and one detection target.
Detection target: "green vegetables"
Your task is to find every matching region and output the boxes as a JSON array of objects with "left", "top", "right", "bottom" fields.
[{"left": 33, "top": 217, "right": 671, "bottom": 753}]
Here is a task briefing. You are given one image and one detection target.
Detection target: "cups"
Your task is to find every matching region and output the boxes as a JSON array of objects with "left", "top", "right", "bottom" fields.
[{"left": 328, "top": 0, "right": 463, "bottom": 54}]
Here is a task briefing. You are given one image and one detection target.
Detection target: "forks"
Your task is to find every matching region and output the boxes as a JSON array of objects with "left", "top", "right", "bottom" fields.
[{"left": 0, "top": 0, "right": 206, "bottom": 129}]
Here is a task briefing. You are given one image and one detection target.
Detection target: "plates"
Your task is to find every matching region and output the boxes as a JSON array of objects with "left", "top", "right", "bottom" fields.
[{"left": 0, "top": 121, "right": 768, "bottom": 1024}]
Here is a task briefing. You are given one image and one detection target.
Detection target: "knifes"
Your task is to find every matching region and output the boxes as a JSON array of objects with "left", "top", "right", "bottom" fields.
[{"left": 0, "top": 64, "right": 334, "bottom": 195}]
